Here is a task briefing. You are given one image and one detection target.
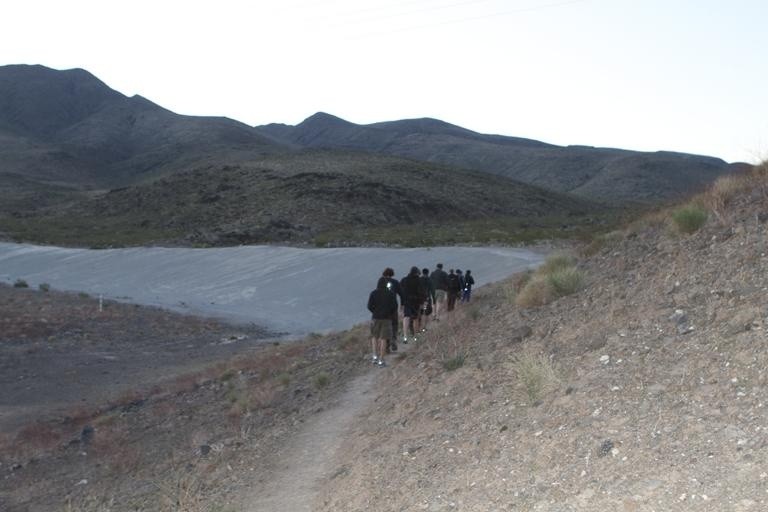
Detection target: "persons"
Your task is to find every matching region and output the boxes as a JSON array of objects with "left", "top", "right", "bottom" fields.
[{"left": 365, "top": 262, "right": 475, "bottom": 368}]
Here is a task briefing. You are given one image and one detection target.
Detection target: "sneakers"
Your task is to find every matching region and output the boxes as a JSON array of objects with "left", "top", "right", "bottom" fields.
[
  {"left": 378, "top": 359, "right": 385, "bottom": 368},
  {"left": 372, "top": 356, "right": 377, "bottom": 363},
  {"left": 391, "top": 341, "right": 398, "bottom": 351}
]
